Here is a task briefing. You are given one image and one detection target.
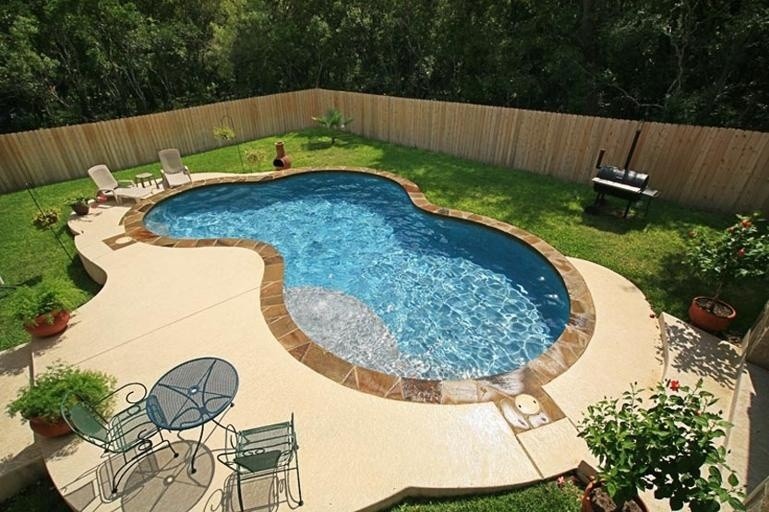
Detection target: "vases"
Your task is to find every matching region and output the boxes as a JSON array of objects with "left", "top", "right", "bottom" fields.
[
  {"left": 685, "top": 295, "right": 738, "bottom": 334},
  {"left": 580, "top": 477, "right": 651, "bottom": 512}
]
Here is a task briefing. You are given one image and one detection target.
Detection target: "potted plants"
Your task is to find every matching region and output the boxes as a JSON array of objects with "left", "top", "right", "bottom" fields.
[
  {"left": 1, "top": 360, "right": 121, "bottom": 441},
  {"left": 62, "top": 189, "right": 98, "bottom": 216},
  {"left": 5, "top": 272, "right": 88, "bottom": 339}
]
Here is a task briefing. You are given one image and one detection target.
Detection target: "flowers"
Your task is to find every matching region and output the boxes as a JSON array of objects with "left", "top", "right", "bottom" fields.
[
  {"left": 574, "top": 373, "right": 756, "bottom": 511},
  {"left": 683, "top": 209, "right": 769, "bottom": 306}
]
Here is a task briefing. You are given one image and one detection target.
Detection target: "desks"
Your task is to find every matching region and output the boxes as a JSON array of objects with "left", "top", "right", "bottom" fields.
[{"left": 145, "top": 357, "right": 251, "bottom": 477}]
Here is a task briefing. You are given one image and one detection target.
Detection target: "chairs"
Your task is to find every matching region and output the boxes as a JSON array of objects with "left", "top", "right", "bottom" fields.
[
  {"left": 216, "top": 412, "right": 304, "bottom": 512},
  {"left": 57, "top": 381, "right": 178, "bottom": 495},
  {"left": 87, "top": 148, "right": 194, "bottom": 205}
]
